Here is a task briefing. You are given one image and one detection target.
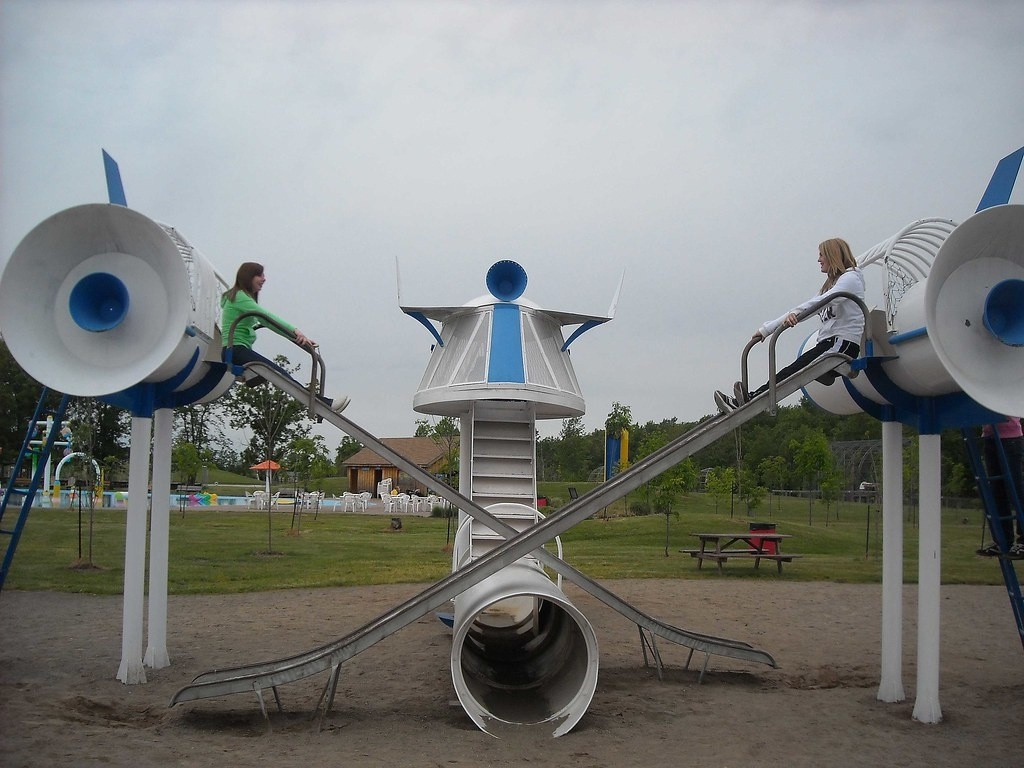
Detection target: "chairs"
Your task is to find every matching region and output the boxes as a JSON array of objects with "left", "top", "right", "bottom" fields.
[{"left": 245, "top": 478, "right": 454, "bottom": 514}]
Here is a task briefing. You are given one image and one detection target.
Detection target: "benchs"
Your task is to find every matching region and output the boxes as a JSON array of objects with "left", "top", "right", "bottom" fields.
[
  {"left": 92, "top": 480, "right": 110, "bottom": 487},
  {"left": 678, "top": 549, "right": 770, "bottom": 570},
  {"left": 697, "top": 553, "right": 803, "bottom": 577},
  {"left": 14, "top": 478, "right": 32, "bottom": 484},
  {"left": 176, "top": 485, "right": 202, "bottom": 493}
]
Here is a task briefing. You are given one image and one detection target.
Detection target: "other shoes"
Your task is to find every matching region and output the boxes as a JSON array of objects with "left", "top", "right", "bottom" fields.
[
  {"left": 331, "top": 396, "right": 347, "bottom": 411},
  {"left": 337, "top": 399, "right": 351, "bottom": 413}
]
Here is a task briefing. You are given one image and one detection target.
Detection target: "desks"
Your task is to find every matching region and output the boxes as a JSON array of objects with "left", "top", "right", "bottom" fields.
[{"left": 688, "top": 533, "right": 792, "bottom": 576}]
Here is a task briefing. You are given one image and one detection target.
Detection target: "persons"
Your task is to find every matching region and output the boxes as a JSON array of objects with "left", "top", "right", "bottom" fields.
[
  {"left": 714, "top": 238, "right": 868, "bottom": 413},
  {"left": 976, "top": 413, "right": 1024, "bottom": 561},
  {"left": 221, "top": 261, "right": 350, "bottom": 414},
  {"left": 390, "top": 486, "right": 401, "bottom": 496}
]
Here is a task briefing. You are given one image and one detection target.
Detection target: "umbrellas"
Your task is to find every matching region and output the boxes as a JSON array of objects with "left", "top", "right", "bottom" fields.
[{"left": 250, "top": 458, "right": 280, "bottom": 493}]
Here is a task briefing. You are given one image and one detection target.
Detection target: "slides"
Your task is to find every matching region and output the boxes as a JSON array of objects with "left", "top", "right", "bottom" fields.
[
  {"left": 239, "top": 353, "right": 782, "bottom": 672},
  {"left": 167, "top": 349, "right": 856, "bottom": 711}
]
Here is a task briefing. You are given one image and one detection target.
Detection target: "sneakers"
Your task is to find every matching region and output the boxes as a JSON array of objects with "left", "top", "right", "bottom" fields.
[
  {"left": 734, "top": 381, "right": 749, "bottom": 407},
  {"left": 714, "top": 390, "right": 738, "bottom": 414}
]
